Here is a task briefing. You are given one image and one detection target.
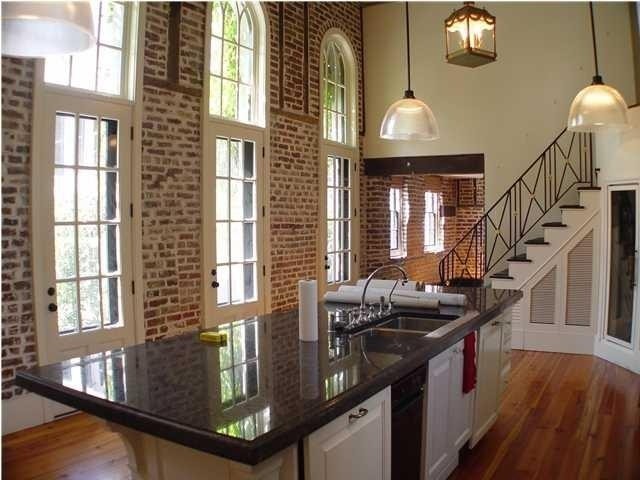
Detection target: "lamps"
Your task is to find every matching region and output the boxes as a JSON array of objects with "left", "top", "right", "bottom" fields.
[
  {"left": 379, "top": 1, "right": 440, "bottom": 141},
  {"left": 443, "top": 2, "right": 498, "bottom": 70},
  {"left": 567, "top": 2, "right": 632, "bottom": 132},
  {"left": 1, "top": 2, "right": 96, "bottom": 59}
]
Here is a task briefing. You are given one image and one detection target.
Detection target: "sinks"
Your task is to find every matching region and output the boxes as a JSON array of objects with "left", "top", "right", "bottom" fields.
[{"left": 375, "top": 312, "right": 461, "bottom": 334}]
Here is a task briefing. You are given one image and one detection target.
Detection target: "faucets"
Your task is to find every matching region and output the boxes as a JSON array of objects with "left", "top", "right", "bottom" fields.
[
  {"left": 360, "top": 334, "right": 382, "bottom": 372},
  {"left": 357, "top": 264, "right": 409, "bottom": 323}
]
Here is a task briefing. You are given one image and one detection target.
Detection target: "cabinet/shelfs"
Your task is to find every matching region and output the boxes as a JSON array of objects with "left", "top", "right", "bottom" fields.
[
  {"left": 420, "top": 304, "right": 513, "bottom": 479},
  {"left": 303, "top": 384, "right": 392, "bottom": 479}
]
